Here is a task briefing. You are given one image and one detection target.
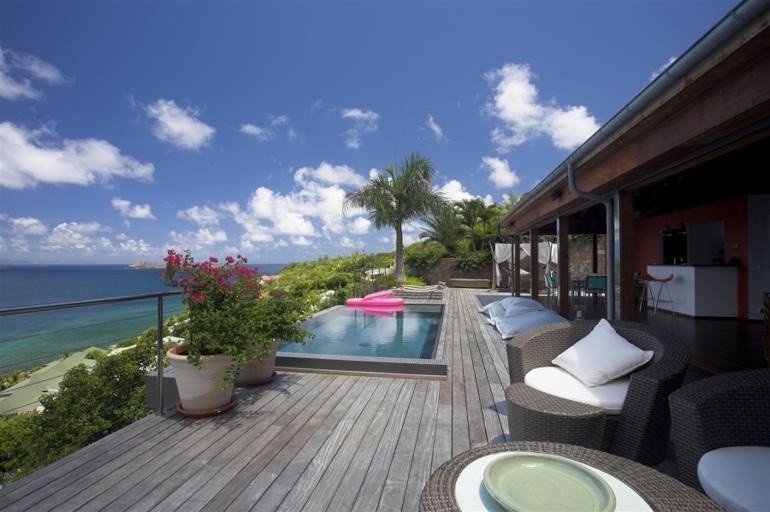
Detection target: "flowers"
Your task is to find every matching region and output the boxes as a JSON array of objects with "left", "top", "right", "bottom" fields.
[
  {"left": 232, "top": 263, "right": 317, "bottom": 363},
  {"left": 160, "top": 248, "right": 262, "bottom": 391}
]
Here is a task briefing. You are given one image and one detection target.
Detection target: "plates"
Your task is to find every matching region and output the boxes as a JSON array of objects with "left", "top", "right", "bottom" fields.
[{"left": 480, "top": 453, "right": 620, "bottom": 512}]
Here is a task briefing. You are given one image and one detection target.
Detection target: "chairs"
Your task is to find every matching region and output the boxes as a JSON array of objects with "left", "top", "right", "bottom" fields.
[
  {"left": 638, "top": 262, "right": 679, "bottom": 318},
  {"left": 580, "top": 274, "right": 607, "bottom": 316},
  {"left": 544, "top": 274, "right": 564, "bottom": 306},
  {"left": 393, "top": 282, "right": 447, "bottom": 300}
]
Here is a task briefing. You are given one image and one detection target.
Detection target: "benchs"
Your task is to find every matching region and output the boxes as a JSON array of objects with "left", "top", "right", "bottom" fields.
[{"left": 451, "top": 278, "right": 491, "bottom": 288}]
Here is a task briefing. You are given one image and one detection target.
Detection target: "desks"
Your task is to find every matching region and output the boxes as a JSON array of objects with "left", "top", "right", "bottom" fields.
[
  {"left": 568, "top": 280, "right": 594, "bottom": 315},
  {"left": 419, "top": 440, "right": 729, "bottom": 512}
]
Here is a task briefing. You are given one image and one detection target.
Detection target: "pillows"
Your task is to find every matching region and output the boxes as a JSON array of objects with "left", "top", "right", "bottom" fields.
[
  {"left": 552, "top": 318, "right": 651, "bottom": 388},
  {"left": 477, "top": 297, "right": 567, "bottom": 339}
]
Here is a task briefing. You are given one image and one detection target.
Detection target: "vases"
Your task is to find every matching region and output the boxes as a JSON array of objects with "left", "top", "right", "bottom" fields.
[
  {"left": 165, "top": 344, "right": 238, "bottom": 416},
  {"left": 235, "top": 335, "right": 280, "bottom": 387}
]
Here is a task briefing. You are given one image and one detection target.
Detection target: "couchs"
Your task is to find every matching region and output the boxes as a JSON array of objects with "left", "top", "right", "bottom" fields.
[
  {"left": 668, "top": 369, "right": 769, "bottom": 512},
  {"left": 504, "top": 320, "right": 691, "bottom": 464}
]
